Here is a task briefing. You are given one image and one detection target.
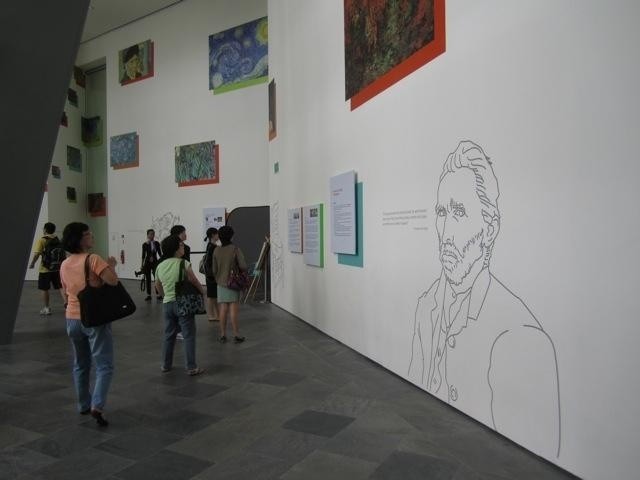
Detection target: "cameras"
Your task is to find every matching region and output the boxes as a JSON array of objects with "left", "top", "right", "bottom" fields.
[{"left": 135, "top": 269, "right": 143, "bottom": 277}]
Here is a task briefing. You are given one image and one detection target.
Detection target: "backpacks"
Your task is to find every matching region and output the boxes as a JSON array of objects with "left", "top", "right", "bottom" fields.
[{"left": 40, "top": 236, "right": 67, "bottom": 271}]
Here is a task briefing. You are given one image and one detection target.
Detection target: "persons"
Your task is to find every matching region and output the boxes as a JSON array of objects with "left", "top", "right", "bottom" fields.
[
  {"left": 139, "top": 228, "right": 164, "bottom": 301},
  {"left": 29, "top": 221, "right": 69, "bottom": 316},
  {"left": 119, "top": 43, "right": 143, "bottom": 84},
  {"left": 59, "top": 221, "right": 119, "bottom": 428},
  {"left": 407, "top": 141, "right": 562, "bottom": 463},
  {"left": 212, "top": 225, "right": 249, "bottom": 345},
  {"left": 199, "top": 226, "right": 223, "bottom": 321},
  {"left": 155, "top": 236, "right": 205, "bottom": 376},
  {"left": 168, "top": 224, "right": 191, "bottom": 264}
]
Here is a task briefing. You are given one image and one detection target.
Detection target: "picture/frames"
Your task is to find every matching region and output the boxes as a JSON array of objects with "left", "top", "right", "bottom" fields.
[
  {"left": 110, "top": 132, "right": 137, "bottom": 167},
  {"left": 208, "top": 15, "right": 269, "bottom": 90},
  {"left": 175, "top": 140, "right": 215, "bottom": 184},
  {"left": 343, "top": 0, "right": 435, "bottom": 102},
  {"left": 119, "top": 38, "right": 149, "bottom": 83},
  {"left": 269, "top": 81, "right": 278, "bottom": 139}
]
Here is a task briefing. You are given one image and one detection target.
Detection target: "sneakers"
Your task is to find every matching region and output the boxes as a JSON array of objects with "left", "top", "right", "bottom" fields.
[
  {"left": 41, "top": 308, "right": 51, "bottom": 314},
  {"left": 188, "top": 368, "right": 204, "bottom": 375},
  {"left": 64, "top": 301, "right": 69, "bottom": 308},
  {"left": 161, "top": 367, "right": 170, "bottom": 372}
]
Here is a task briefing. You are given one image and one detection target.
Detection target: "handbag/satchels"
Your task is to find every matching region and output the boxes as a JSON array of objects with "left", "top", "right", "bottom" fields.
[
  {"left": 199, "top": 253, "right": 208, "bottom": 272},
  {"left": 78, "top": 254, "right": 137, "bottom": 328},
  {"left": 227, "top": 244, "right": 248, "bottom": 289},
  {"left": 175, "top": 263, "right": 207, "bottom": 318}
]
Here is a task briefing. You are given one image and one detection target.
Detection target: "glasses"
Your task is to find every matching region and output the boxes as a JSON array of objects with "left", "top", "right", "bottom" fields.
[{"left": 82, "top": 232, "right": 95, "bottom": 237}]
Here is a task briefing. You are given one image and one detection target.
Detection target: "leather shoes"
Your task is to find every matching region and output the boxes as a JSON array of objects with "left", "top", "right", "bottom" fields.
[
  {"left": 145, "top": 296, "right": 151, "bottom": 301},
  {"left": 90, "top": 409, "right": 109, "bottom": 427},
  {"left": 80, "top": 410, "right": 89, "bottom": 414},
  {"left": 217, "top": 337, "right": 226, "bottom": 342},
  {"left": 156, "top": 296, "right": 162, "bottom": 299},
  {"left": 234, "top": 335, "right": 245, "bottom": 341}
]
